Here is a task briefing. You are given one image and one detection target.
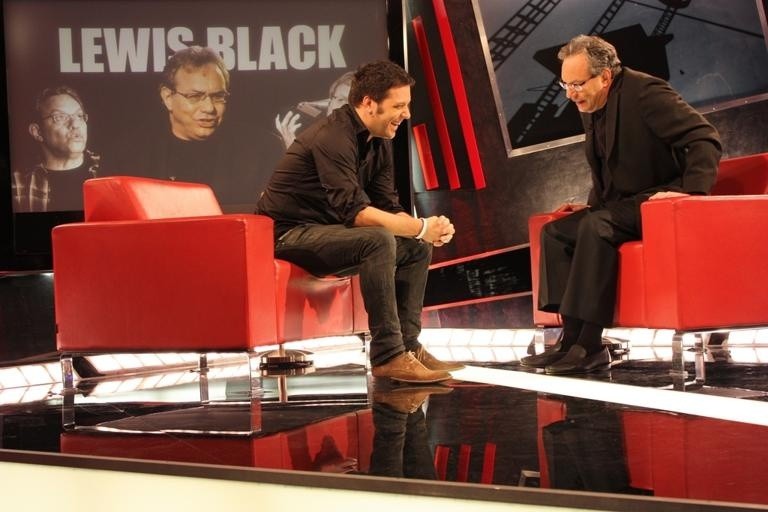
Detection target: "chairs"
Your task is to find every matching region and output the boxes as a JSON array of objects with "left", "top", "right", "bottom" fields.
[
  {"left": 527, "top": 153, "right": 766, "bottom": 369},
  {"left": 533, "top": 368, "right": 765, "bottom": 506},
  {"left": 50, "top": 175, "right": 370, "bottom": 389},
  {"left": 59, "top": 389, "right": 374, "bottom": 474}
]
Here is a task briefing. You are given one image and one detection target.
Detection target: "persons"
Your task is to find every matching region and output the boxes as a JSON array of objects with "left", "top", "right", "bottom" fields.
[
  {"left": 9, "top": 83, "right": 120, "bottom": 213},
  {"left": 123, "top": 45, "right": 290, "bottom": 209},
  {"left": 516, "top": 31, "right": 721, "bottom": 376},
  {"left": 251, "top": 57, "right": 469, "bottom": 385},
  {"left": 273, "top": 69, "right": 354, "bottom": 152}
]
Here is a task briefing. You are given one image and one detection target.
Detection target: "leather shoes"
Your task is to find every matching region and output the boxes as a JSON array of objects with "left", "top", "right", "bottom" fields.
[
  {"left": 520, "top": 341, "right": 566, "bottom": 368},
  {"left": 547, "top": 346, "right": 613, "bottom": 376},
  {"left": 372, "top": 350, "right": 454, "bottom": 383},
  {"left": 408, "top": 345, "right": 466, "bottom": 370},
  {"left": 374, "top": 384, "right": 454, "bottom": 414}
]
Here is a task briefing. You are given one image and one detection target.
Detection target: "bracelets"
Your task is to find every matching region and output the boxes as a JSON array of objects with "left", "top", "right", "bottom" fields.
[{"left": 412, "top": 216, "right": 428, "bottom": 242}]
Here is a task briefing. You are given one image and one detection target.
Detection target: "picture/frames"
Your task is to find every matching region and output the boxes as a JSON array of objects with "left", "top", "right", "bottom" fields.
[{"left": 468, "top": 0, "right": 768, "bottom": 161}]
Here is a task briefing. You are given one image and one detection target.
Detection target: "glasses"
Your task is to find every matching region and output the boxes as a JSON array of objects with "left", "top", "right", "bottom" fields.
[
  {"left": 333, "top": 95, "right": 346, "bottom": 107},
  {"left": 39, "top": 113, "right": 88, "bottom": 124},
  {"left": 177, "top": 90, "right": 230, "bottom": 104},
  {"left": 557, "top": 76, "right": 598, "bottom": 93}
]
